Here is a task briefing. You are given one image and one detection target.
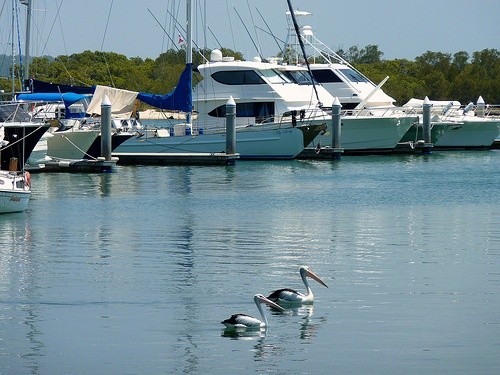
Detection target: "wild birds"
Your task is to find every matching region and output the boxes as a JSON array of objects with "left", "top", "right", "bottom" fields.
[
  {"left": 266, "top": 266, "right": 328, "bottom": 305},
  {"left": 220, "top": 294, "right": 286, "bottom": 330}
]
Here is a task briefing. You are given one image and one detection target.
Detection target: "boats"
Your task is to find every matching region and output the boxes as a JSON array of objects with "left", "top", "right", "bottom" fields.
[{"left": 0, "top": 0, "right": 500, "bottom": 213}]
